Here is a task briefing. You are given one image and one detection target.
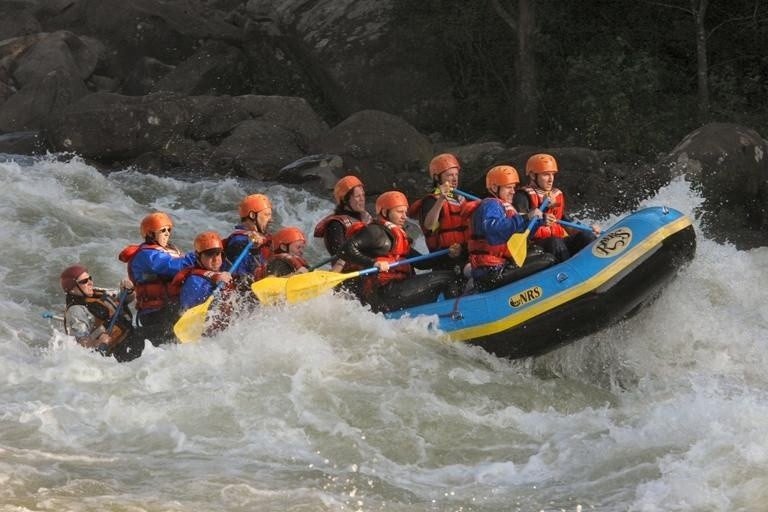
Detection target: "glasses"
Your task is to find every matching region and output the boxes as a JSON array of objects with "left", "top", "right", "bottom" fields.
[
  {"left": 203, "top": 249, "right": 220, "bottom": 256},
  {"left": 78, "top": 275, "right": 92, "bottom": 284},
  {"left": 160, "top": 228, "right": 171, "bottom": 233}
]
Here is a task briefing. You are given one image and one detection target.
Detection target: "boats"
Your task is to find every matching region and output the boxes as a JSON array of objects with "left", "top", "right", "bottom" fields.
[{"left": 153, "top": 205, "right": 695, "bottom": 357}]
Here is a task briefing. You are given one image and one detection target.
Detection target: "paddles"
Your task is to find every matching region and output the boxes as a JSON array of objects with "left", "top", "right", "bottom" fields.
[
  {"left": 284, "top": 242, "right": 461, "bottom": 304},
  {"left": 174, "top": 237, "right": 258, "bottom": 344},
  {"left": 507, "top": 196, "right": 553, "bottom": 269},
  {"left": 251, "top": 254, "right": 339, "bottom": 306}
]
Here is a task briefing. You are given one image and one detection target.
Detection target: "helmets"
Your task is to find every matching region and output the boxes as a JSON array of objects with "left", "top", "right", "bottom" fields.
[
  {"left": 376, "top": 190, "right": 409, "bottom": 215},
  {"left": 525, "top": 154, "right": 558, "bottom": 176},
  {"left": 271, "top": 228, "right": 305, "bottom": 251},
  {"left": 430, "top": 153, "right": 461, "bottom": 179},
  {"left": 240, "top": 194, "right": 272, "bottom": 217},
  {"left": 61, "top": 266, "right": 88, "bottom": 292},
  {"left": 194, "top": 232, "right": 223, "bottom": 253},
  {"left": 141, "top": 212, "right": 172, "bottom": 238},
  {"left": 334, "top": 176, "right": 364, "bottom": 204},
  {"left": 486, "top": 165, "right": 520, "bottom": 188}
]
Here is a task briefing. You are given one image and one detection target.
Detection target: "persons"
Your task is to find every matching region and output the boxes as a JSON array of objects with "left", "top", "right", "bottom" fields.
[
  {"left": 60, "top": 262, "right": 146, "bottom": 364},
  {"left": 336, "top": 190, "right": 463, "bottom": 311},
  {"left": 117, "top": 211, "right": 195, "bottom": 349},
  {"left": 178, "top": 229, "right": 263, "bottom": 312},
  {"left": 219, "top": 193, "right": 274, "bottom": 282},
  {"left": 253, "top": 226, "right": 351, "bottom": 281},
  {"left": 471, "top": 164, "right": 559, "bottom": 292},
  {"left": 314, "top": 175, "right": 374, "bottom": 294},
  {"left": 512, "top": 152, "right": 598, "bottom": 264},
  {"left": 419, "top": 152, "right": 481, "bottom": 270}
]
